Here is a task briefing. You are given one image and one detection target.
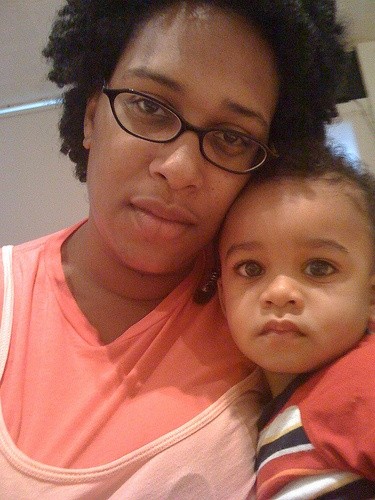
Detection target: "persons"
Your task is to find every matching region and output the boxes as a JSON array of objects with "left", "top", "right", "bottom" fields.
[
  {"left": 205, "top": 129, "right": 375, "bottom": 500},
  {"left": 0, "top": 0, "right": 351, "bottom": 500}
]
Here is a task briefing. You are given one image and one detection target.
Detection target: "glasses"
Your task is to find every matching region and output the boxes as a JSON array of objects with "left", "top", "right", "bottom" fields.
[{"left": 101, "top": 75, "right": 280, "bottom": 174}]
